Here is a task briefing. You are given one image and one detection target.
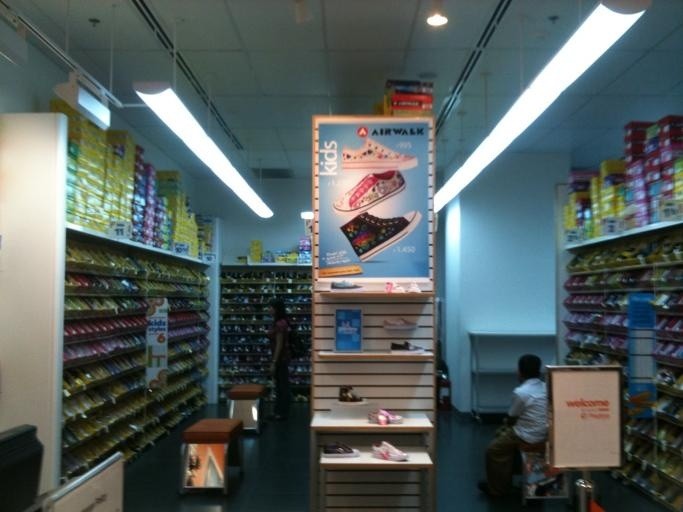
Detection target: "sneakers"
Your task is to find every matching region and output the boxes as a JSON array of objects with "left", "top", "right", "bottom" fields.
[
  {"left": 339, "top": 211, "right": 422, "bottom": 263},
  {"left": 392, "top": 341, "right": 424, "bottom": 354},
  {"left": 339, "top": 386, "right": 366, "bottom": 404},
  {"left": 372, "top": 440, "right": 408, "bottom": 460},
  {"left": 323, "top": 441, "right": 360, "bottom": 457},
  {"left": 342, "top": 138, "right": 417, "bottom": 171},
  {"left": 478, "top": 481, "right": 523, "bottom": 504},
  {"left": 333, "top": 170, "right": 406, "bottom": 214},
  {"left": 384, "top": 318, "right": 417, "bottom": 332},
  {"left": 368, "top": 409, "right": 403, "bottom": 424}
]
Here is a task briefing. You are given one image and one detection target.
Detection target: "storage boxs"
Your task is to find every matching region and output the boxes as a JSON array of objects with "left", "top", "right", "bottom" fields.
[
  {"left": 519, "top": 442, "right": 572, "bottom": 500},
  {"left": 545, "top": 364, "right": 625, "bottom": 471},
  {"left": 563, "top": 115, "right": 683, "bottom": 245},
  {"left": 44, "top": 100, "right": 216, "bottom": 263}
]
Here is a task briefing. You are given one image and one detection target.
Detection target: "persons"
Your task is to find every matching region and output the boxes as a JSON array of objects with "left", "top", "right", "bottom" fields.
[
  {"left": 478, "top": 354, "right": 549, "bottom": 501},
  {"left": 267, "top": 297, "right": 292, "bottom": 424}
]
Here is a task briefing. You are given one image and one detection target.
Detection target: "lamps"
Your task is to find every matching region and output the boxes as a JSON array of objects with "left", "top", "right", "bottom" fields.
[
  {"left": 434, "top": 1, "right": 654, "bottom": 214},
  {"left": 425, "top": 1, "right": 448, "bottom": 27},
  {"left": 53, "top": 68, "right": 113, "bottom": 130},
  {"left": 131, "top": 16, "right": 275, "bottom": 219}
]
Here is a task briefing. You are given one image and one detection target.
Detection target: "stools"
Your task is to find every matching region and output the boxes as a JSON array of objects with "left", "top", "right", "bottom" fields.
[
  {"left": 227, "top": 383, "right": 265, "bottom": 435},
  {"left": 181, "top": 419, "right": 243, "bottom": 512}
]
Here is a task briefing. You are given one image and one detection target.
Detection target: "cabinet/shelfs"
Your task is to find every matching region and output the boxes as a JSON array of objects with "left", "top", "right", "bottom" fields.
[
  {"left": 0, "top": 111, "right": 210, "bottom": 486},
  {"left": 219, "top": 260, "right": 312, "bottom": 413},
  {"left": 469, "top": 330, "right": 559, "bottom": 421},
  {"left": 553, "top": 179, "right": 683, "bottom": 511},
  {"left": 310, "top": 286, "right": 436, "bottom": 512}
]
